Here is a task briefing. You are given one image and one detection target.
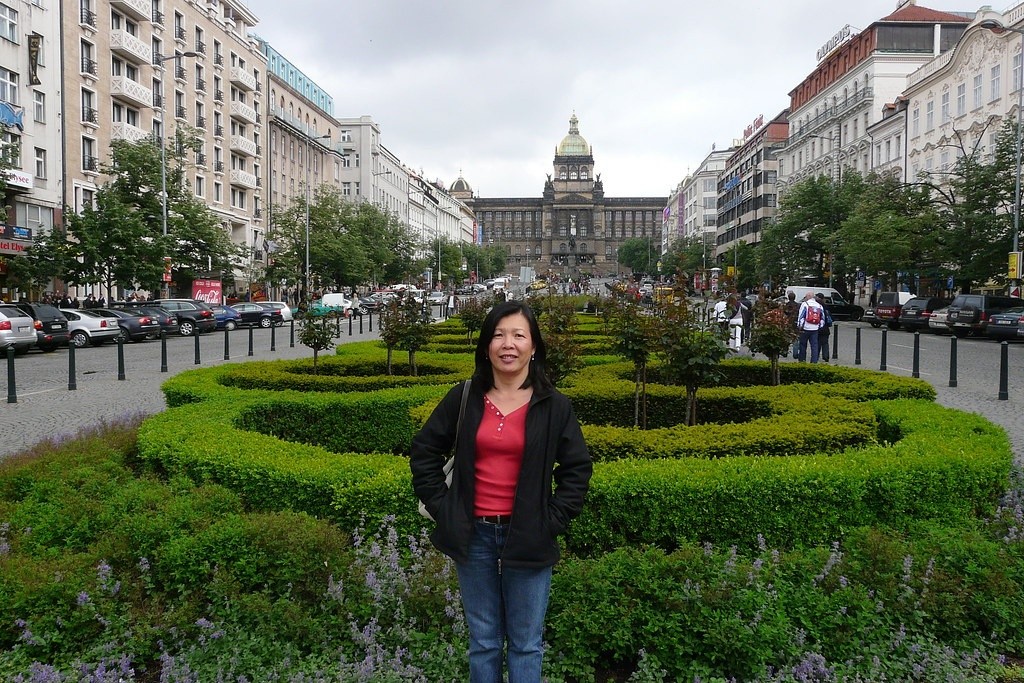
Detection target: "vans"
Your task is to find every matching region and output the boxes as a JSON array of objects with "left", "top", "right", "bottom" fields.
[{"left": 785, "top": 287, "right": 865, "bottom": 322}]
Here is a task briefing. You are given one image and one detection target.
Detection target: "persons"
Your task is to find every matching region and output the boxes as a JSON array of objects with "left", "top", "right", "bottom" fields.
[
  {"left": 3, "top": 290, "right": 155, "bottom": 309},
  {"left": 281, "top": 271, "right": 832, "bottom": 364},
  {"left": 408, "top": 300, "right": 592, "bottom": 683}
]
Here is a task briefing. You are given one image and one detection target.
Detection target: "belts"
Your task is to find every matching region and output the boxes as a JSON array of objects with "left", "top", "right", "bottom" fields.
[{"left": 477, "top": 515, "right": 511, "bottom": 525}]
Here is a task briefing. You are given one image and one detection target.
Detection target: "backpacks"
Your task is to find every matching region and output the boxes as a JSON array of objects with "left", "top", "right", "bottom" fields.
[
  {"left": 805, "top": 301, "right": 822, "bottom": 324},
  {"left": 824, "top": 310, "right": 832, "bottom": 327}
]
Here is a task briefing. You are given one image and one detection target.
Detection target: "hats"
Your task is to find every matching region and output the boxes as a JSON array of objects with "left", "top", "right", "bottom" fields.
[{"left": 815, "top": 293, "right": 824, "bottom": 299}]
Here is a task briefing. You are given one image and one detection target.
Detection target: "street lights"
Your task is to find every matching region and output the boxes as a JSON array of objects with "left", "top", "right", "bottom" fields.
[
  {"left": 407, "top": 178, "right": 423, "bottom": 287},
  {"left": 810, "top": 123, "right": 841, "bottom": 184},
  {"left": 162, "top": 51, "right": 197, "bottom": 258},
  {"left": 438, "top": 206, "right": 452, "bottom": 292},
  {"left": 693, "top": 204, "right": 707, "bottom": 269},
  {"left": 372, "top": 171, "right": 392, "bottom": 204},
  {"left": 305, "top": 133, "right": 332, "bottom": 293}
]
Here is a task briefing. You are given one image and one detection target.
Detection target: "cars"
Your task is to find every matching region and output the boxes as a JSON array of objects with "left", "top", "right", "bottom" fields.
[
  {"left": 210, "top": 305, "right": 243, "bottom": 331},
  {"left": 129, "top": 307, "right": 181, "bottom": 341},
  {"left": 532, "top": 281, "right": 546, "bottom": 290},
  {"left": 861, "top": 291, "right": 1024, "bottom": 342},
  {"left": 291, "top": 273, "right": 512, "bottom": 321},
  {"left": 59, "top": 308, "right": 121, "bottom": 347},
  {"left": 612, "top": 273, "right": 674, "bottom": 306},
  {"left": 85, "top": 308, "right": 163, "bottom": 346},
  {"left": 229, "top": 303, "right": 282, "bottom": 328}
]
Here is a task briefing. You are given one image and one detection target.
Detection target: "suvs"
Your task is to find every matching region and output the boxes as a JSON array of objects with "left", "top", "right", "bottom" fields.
[
  {"left": 17, "top": 303, "right": 71, "bottom": 354},
  {"left": 0, "top": 303, "right": 38, "bottom": 357},
  {"left": 257, "top": 301, "right": 293, "bottom": 327},
  {"left": 140, "top": 299, "right": 216, "bottom": 337}
]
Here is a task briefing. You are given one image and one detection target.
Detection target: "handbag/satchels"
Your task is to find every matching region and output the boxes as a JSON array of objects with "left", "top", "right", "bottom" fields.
[
  {"left": 418, "top": 379, "right": 473, "bottom": 520},
  {"left": 793, "top": 341, "right": 800, "bottom": 359}
]
